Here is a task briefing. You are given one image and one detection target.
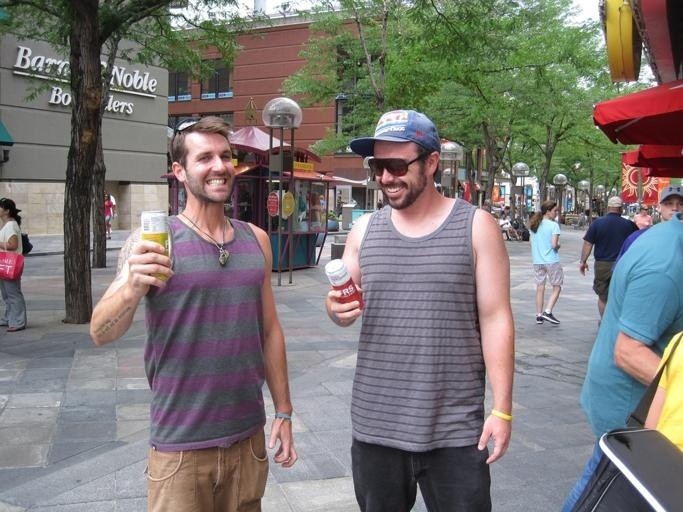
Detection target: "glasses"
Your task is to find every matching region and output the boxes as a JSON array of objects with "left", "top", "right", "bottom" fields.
[
  {"left": 368, "top": 154, "right": 431, "bottom": 176},
  {"left": 170, "top": 117, "right": 233, "bottom": 162},
  {"left": 642, "top": 208, "right": 648, "bottom": 210},
  {"left": 104, "top": 195, "right": 108, "bottom": 197}
]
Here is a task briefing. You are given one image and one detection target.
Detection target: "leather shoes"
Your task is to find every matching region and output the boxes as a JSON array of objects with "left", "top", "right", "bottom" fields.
[{"left": 7, "top": 325, "right": 18, "bottom": 331}]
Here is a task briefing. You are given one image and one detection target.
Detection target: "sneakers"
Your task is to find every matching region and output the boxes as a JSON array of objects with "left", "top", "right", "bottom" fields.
[
  {"left": 542, "top": 311, "right": 561, "bottom": 324},
  {"left": 537, "top": 314, "right": 543, "bottom": 324}
]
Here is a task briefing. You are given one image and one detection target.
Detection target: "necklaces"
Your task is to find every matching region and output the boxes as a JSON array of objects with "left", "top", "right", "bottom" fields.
[{"left": 181, "top": 215, "right": 229, "bottom": 266}]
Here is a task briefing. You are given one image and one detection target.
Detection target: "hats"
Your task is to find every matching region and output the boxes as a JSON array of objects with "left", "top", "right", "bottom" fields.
[
  {"left": 350, "top": 109, "right": 442, "bottom": 155},
  {"left": 608, "top": 196, "right": 623, "bottom": 207},
  {"left": 1, "top": 196, "right": 17, "bottom": 212},
  {"left": 660, "top": 185, "right": 683, "bottom": 202}
]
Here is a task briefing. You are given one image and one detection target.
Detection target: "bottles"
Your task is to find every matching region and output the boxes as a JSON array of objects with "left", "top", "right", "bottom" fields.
[
  {"left": 323, "top": 259, "right": 364, "bottom": 313},
  {"left": 140, "top": 210, "right": 170, "bottom": 283}
]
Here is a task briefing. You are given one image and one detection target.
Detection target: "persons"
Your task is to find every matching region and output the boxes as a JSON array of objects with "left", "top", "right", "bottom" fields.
[
  {"left": 498, "top": 211, "right": 519, "bottom": 240},
  {"left": 645, "top": 330, "right": 683, "bottom": 457},
  {"left": 499, "top": 203, "right": 512, "bottom": 240},
  {"left": 530, "top": 201, "right": 562, "bottom": 324},
  {"left": 376, "top": 197, "right": 382, "bottom": 211},
  {"left": 104, "top": 193, "right": 114, "bottom": 239},
  {"left": 90, "top": 117, "right": 296, "bottom": 512},
  {"left": 104, "top": 190, "right": 116, "bottom": 234},
  {"left": 611, "top": 186, "right": 683, "bottom": 276},
  {"left": 562, "top": 211, "right": 682, "bottom": 511},
  {"left": 580, "top": 196, "right": 639, "bottom": 326},
  {"left": 337, "top": 196, "right": 345, "bottom": 219},
  {"left": 632, "top": 202, "right": 653, "bottom": 231},
  {"left": 327, "top": 110, "right": 516, "bottom": 512},
  {"left": 0, "top": 199, "right": 26, "bottom": 332}
]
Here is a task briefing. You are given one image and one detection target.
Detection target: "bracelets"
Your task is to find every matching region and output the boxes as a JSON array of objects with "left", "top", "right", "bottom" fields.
[
  {"left": 275, "top": 413, "right": 291, "bottom": 418},
  {"left": 491, "top": 410, "right": 516, "bottom": 420}
]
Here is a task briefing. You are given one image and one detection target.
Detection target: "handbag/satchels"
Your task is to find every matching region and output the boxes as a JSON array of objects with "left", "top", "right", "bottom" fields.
[
  {"left": 564, "top": 334, "right": 683, "bottom": 511},
  {"left": 21, "top": 232, "right": 33, "bottom": 255},
  {"left": 1, "top": 241, "right": 24, "bottom": 280}
]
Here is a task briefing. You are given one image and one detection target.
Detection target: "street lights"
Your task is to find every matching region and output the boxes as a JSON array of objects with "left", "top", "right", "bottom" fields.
[
  {"left": 594, "top": 185, "right": 604, "bottom": 217},
  {"left": 438, "top": 141, "right": 462, "bottom": 198},
  {"left": 609, "top": 188, "right": 616, "bottom": 197},
  {"left": 261, "top": 97, "right": 301, "bottom": 286},
  {"left": 511, "top": 162, "right": 528, "bottom": 241},
  {"left": 552, "top": 174, "right": 566, "bottom": 230},
  {"left": 577, "top": 180, "right": 589, "bottom": 226}
]
